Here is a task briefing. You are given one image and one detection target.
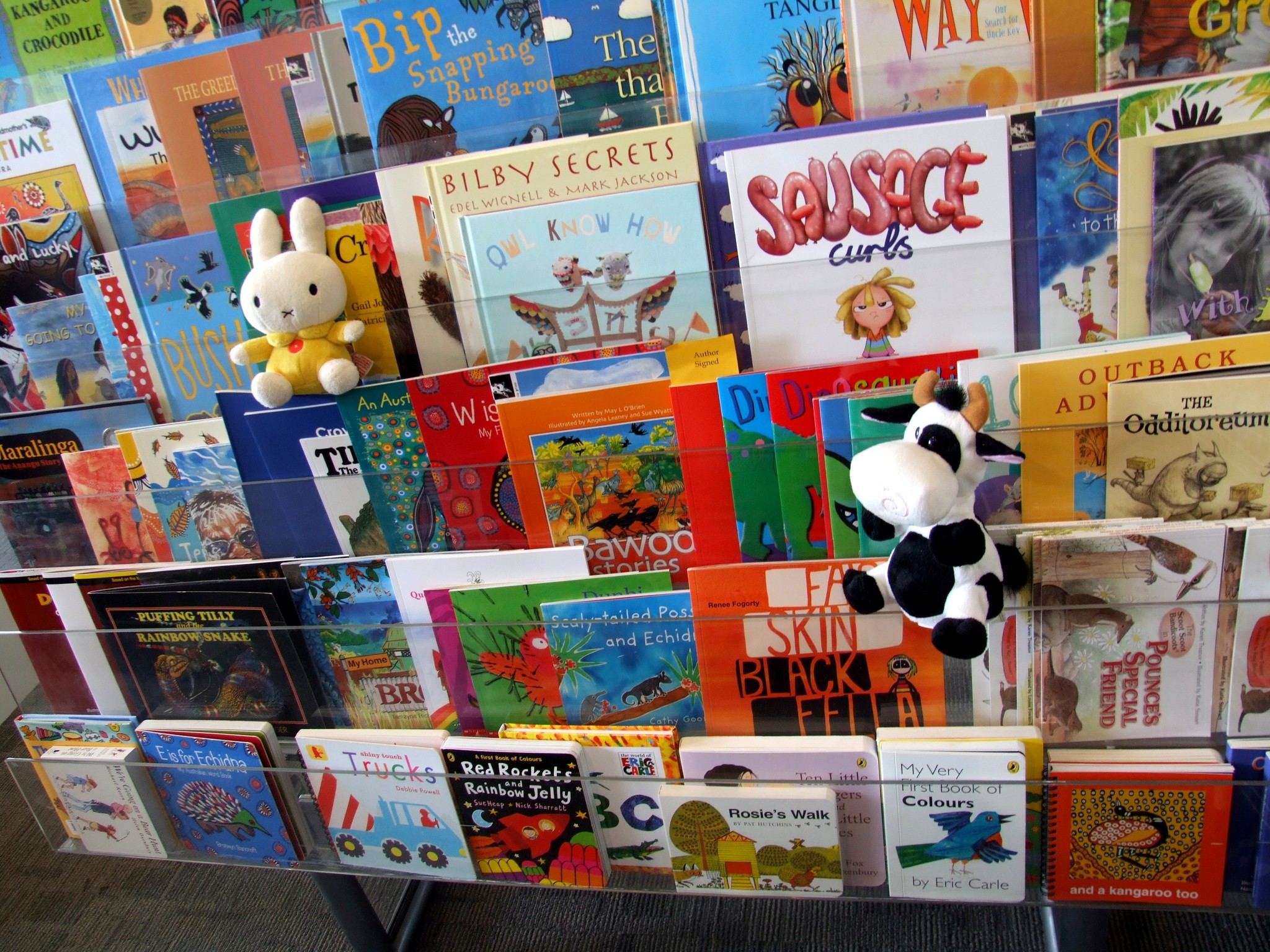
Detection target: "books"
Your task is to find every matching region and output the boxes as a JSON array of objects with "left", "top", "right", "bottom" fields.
[{"left": 0, "top": 0, "right": 1270, "bottom": 912}]
